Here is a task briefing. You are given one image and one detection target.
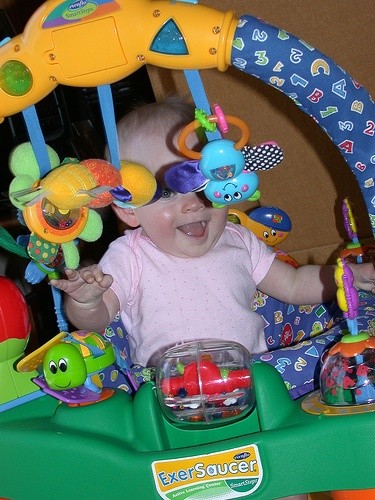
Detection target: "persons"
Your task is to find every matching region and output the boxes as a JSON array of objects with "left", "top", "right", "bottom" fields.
[{"left": 46, "top": 93, "right": 375, "bottom": 398}]
